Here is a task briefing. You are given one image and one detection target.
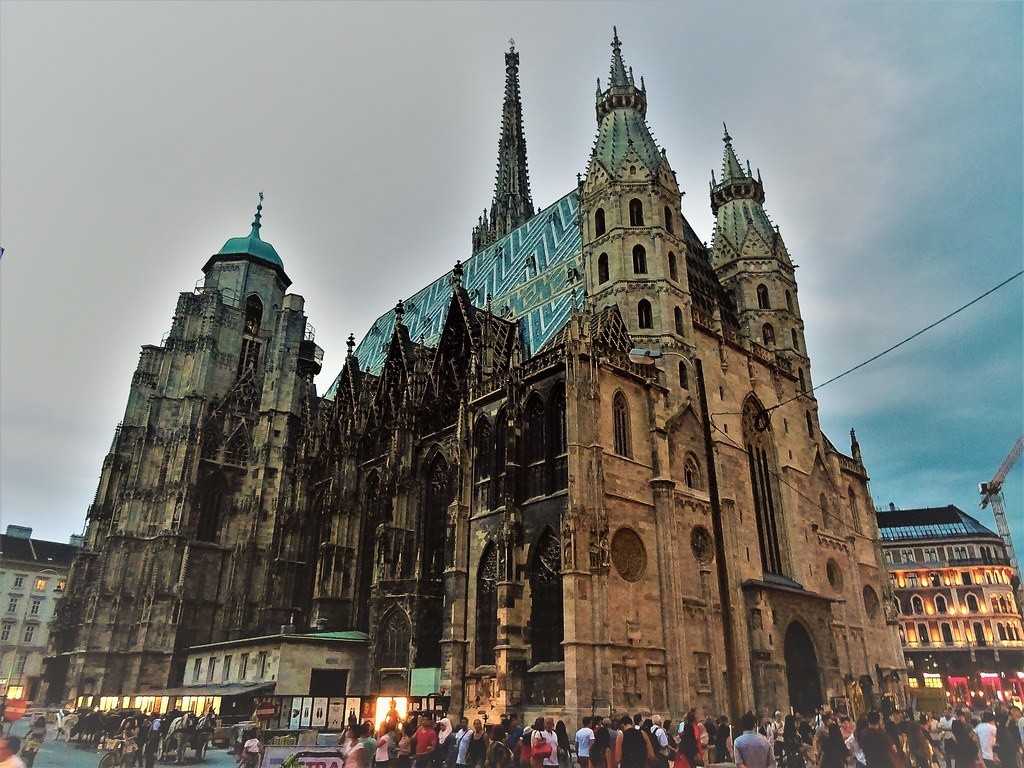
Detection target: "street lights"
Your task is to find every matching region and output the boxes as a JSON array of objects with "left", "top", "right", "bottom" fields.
[{"left": 627, "top": 346, "right": 740, "bottom": 730}]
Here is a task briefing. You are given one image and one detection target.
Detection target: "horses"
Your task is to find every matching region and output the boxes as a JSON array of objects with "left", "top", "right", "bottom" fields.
[{"left": 160, "top": 707, "right": 217, "bottom": 763}]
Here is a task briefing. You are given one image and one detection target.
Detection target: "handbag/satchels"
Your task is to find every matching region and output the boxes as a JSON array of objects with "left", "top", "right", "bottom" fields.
[{"left": 531, "top": 731, "right": 552, "bottom": 758}]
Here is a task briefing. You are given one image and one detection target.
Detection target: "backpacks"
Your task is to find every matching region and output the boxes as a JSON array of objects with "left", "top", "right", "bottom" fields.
[
  {"left": 679, "top": 724, "right": 699, "bottom": 757},
  {"left": 648, "top": 727, "right": 661, "bottom": 756}
]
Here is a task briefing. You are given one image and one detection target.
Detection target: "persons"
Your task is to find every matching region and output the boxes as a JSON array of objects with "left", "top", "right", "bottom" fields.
[{"left": 0, "top": 699, "right": 1024, "bottom": 768}]
